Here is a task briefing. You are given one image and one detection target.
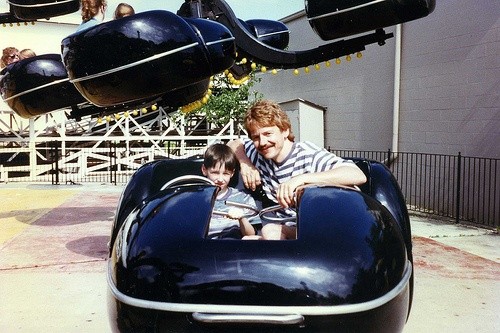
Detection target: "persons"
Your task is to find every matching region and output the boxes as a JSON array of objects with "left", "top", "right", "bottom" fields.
[
  {"left": 77, "top": 0, "right": 109, "bottom": 31},
  {"left": 0, "top": 46, "right": 36, "bottom": 68},
  {"left": 115, "top": 2, "right": 135, "bottom": 20},
  {"left": 226, "top": 100, "right": 367, "bottom": 240},
  {"left": 201, "top": 144, "right": 262, "bottom": 240}
]
